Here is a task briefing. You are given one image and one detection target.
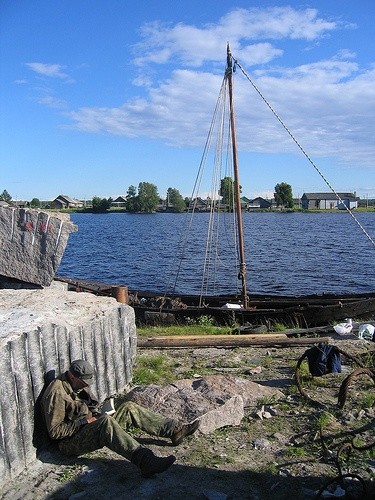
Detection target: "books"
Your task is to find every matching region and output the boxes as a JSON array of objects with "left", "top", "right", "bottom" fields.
[{"left": 95, "top": 397, "right": 117, "bottom": 421}]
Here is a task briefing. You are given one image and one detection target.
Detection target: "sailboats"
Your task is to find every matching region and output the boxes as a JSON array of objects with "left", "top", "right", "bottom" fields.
[{"left": 53, "top": 41, "right": 375, "bottom": 327}]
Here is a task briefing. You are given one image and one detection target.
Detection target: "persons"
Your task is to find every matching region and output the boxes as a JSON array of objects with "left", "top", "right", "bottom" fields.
[{"left": 40, "top": 359, "right": 202, "bottom": 479}]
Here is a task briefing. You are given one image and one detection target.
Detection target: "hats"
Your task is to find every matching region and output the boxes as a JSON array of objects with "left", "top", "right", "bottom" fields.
[{"left": 69, "top": 360, "right": 94, "bottom": 385}]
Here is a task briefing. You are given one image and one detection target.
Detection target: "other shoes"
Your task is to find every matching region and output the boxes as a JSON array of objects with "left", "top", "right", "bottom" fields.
[
  {"left": 142, "top": 455, "right": 176, "bottom": 476},
  {"left": 170, "top": 420, "right": 201, "bottom": 446}
]
